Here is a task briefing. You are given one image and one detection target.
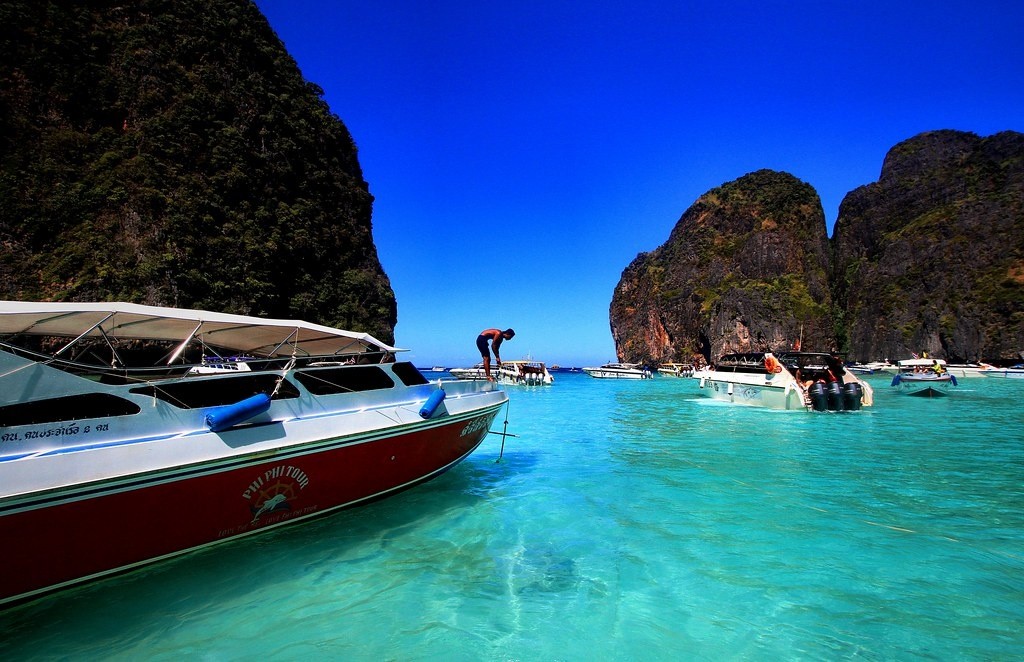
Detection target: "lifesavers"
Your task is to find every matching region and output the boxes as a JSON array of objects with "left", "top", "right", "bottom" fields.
[{"left": 765, "top": 358, "right": 774, "bottom": 373}]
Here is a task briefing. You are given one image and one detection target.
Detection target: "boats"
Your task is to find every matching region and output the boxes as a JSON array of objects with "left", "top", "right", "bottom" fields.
[
  {"left": 431, "top": 365, "right": 445, "bottom": 372},
  {"left": 851, "top": 355, "right": 899, "bottom": 375},
  {"left": 691, "top": 351, "right": 874, "bottom": 414},
  {"left": 893, "top": 359, "right": 954, "bottom": 396},
  {"left": 582, "top": 362, "right": 650, "bottom": 379},
  {"left": 979, "top": 362, "right": 1024, "bottom": 380},
  {"left": 945, "top": 363, "right": 986, "bottom": 378},
  {"left": 0, "top": 301, "right": 510, "bottom": 611},
  {"left": 500, "top": 360, "right": 555, "bottom": 387},
  {"left": 691, "top": 367, "right": 711, "bottom": 379},
  {"left": 657, "top": 362, "right": 693, "bottom": 378},
  {"left": 449, "top": 361, "right": 502, "bottom": 380}
]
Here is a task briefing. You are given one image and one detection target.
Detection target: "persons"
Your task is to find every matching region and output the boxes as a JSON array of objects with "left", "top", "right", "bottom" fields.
[
  {"left": 913, "top": 349, "right": 929, "bottom": 359},
  {"left": 476, "top": 329, "right": 515, "bottom": 382},
  {"left": 930, "top": 360, "right": 942, "bottom": 374},
  {"left": 913, "top": 365, "right": 928, "bottom": 375}
]
